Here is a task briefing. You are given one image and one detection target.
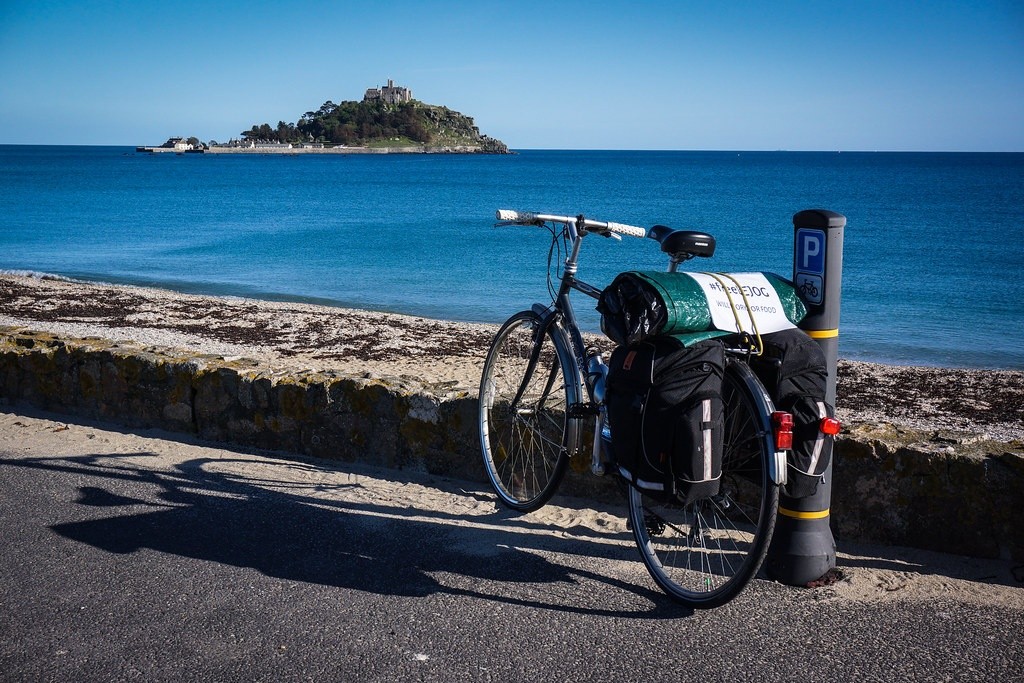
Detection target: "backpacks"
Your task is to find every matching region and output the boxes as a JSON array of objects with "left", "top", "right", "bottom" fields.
[
  {"left": 750, "top": 328, "right": 836, "bottom": 500},
  {"left": 605, "top": 341, "right": 726, "bottom": 500}
]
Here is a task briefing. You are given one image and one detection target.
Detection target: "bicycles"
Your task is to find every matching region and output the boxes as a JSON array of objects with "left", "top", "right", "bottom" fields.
[{"left": 478, "top": 208, "right": 792, "bottom": 608}]
